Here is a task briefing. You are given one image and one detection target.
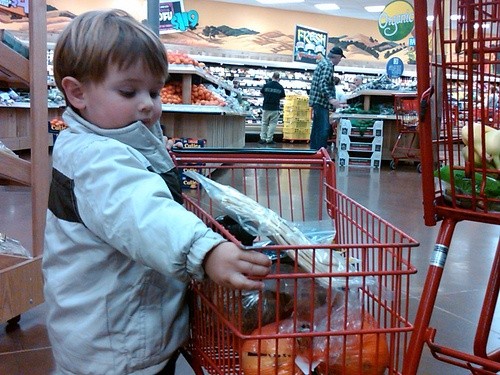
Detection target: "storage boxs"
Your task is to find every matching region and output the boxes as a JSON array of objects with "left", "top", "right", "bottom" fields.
[
  {"left": 335, "top": 119, "right": 383, "bottom": 169},
  {"left": 282, "top": 94, "right": 312, "bottom": 143}
]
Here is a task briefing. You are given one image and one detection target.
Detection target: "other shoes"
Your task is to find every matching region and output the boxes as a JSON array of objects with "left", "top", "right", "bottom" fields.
[
  {"left": 259, "top": 139, "right": 266, "bottom": 143},
  {"left": 266, "top": 141, "right": 277, "bottom": 144}
]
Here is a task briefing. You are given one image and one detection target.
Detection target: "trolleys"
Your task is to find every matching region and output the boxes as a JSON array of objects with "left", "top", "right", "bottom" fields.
[
  {"left": 401, "top": 0, "right": 500, "bottom": 375},
  {"left": 390, "top": 93, "right": 422, "bottom": 174},
  {"left": 166, "top": 146, "right": 421, "bottom": 375}
]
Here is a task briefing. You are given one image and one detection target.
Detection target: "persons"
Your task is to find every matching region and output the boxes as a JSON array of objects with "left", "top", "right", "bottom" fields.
[
  {"left": 308, "top": 46, "right": 346, "bottom": 153},
  {"left": 489, "top": 86, "right": 499, "bottom": 108},
  {"left": 351, "top": 76, "right": 363, "bottom": 91},
  {"left": 334, "top": 76, "right": 344, "bottom": 93},
  {"left": 39, "top": 7, "right": 272, "bottom": 375},
  {"left": 259, "top": 71, "right": 285, "bottom": 145}
]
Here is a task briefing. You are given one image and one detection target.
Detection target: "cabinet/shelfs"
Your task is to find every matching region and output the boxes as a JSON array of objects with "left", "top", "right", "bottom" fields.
[
  {"left": 329, "top": 90, "right": 424, "bottom": 168},
  {"left": 0, "top": 0, "right": 54, "bottom": 326},
  {"left": 162, "top": 64, "right": 246, "bottom": 180}
]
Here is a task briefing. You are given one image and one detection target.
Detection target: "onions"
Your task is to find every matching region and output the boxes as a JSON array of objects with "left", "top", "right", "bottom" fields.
[{"left": 460, "top": 122, "right": 500, "bottom": 174}]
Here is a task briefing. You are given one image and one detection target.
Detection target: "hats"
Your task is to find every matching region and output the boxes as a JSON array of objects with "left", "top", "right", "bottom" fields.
[{"left": 329, "top": 47, "right": 346, "bottom": 58}]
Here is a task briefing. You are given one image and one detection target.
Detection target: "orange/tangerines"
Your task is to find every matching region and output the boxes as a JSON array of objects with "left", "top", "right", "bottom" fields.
[
  {"left": 51, "top": 119, "right": 66, "bottom": 127},
  {"left": 241, "top": 319, "right": 390, "bottom": 375},
  {"left": 166, "top": 52, "right": 199, "bottom": 66},
  {"left": 160, "top": 82, "right": 227, "bottom": 106}
]
